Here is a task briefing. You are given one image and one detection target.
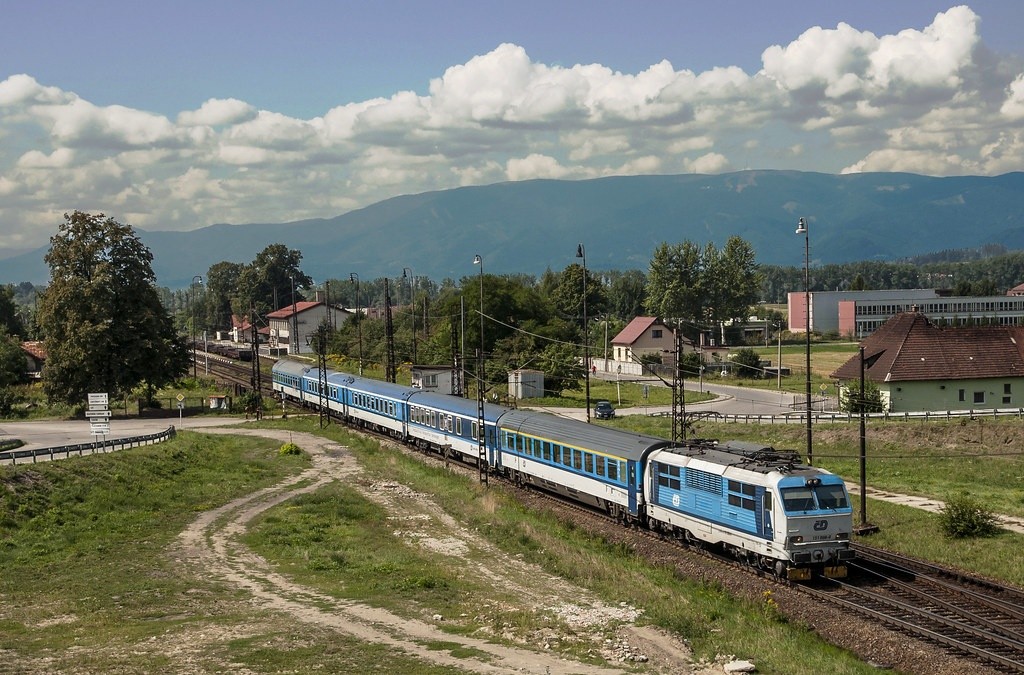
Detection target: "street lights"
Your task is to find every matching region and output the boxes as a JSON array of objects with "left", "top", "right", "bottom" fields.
[
  {"left": 192, "top": 276, "right": 203, "bottom": 380},
  {"left": 772, "top": 321, "right": 781, "bottom": 388},
  {"left": 795, "top": 217, "right": 813, "bottom": 467},
  {"left": 473, "top": 255, "right": 485, "bottom": 391},
  {"left": 576, "top": 243, "right": 591, "bottom": 424},
  {"left": 403, "top": 268, "right": 416, "bottom": 365},
  {"left": 765, "top": 319, "right": 768, "bottom": 347},
  {"left": 350, "top": 273, "right": 363, "bottom": 376}
]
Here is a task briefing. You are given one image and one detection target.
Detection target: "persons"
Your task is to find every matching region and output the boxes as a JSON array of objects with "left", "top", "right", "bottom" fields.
[
  {"left": 256, "top": 406, "right": 263, "bottom": 420},
  {"left": 245, "top": 405, "right": 248, "bottom": 419},
  {"left": 592, "top": 366, "right": 596, "bottom": 373}
]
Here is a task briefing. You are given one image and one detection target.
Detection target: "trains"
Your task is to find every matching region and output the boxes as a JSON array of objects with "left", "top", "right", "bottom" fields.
[
  {"left": 271, "top": 359, "right": 857, "bottom": 580},
  {"left": 190, "top": 338, "right": 253, "bottom": 363}
]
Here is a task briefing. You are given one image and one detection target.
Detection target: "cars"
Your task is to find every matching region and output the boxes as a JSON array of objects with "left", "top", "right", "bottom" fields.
[{"left": 593, "top": 402, "right": 616, "bottom": 419}]
